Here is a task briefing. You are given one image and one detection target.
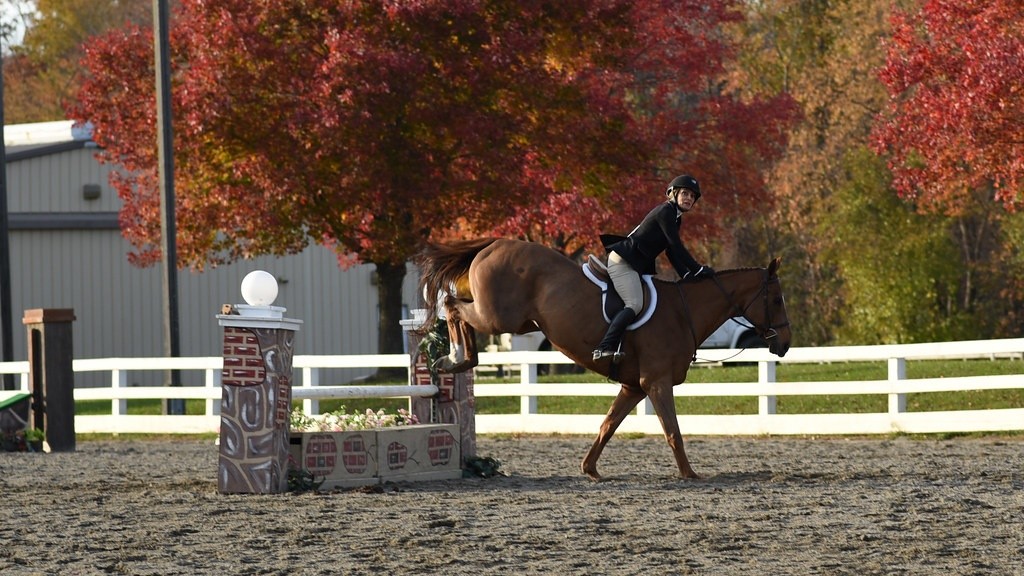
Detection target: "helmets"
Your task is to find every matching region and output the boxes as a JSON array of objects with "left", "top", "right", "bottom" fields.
[{"left": 665, "top": 175, "right": 701, "bottom": 201}]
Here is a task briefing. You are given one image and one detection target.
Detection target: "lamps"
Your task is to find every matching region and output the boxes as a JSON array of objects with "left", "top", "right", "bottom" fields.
[
  {"left": 240, "top": 270, "right": 279, "bottom": 307},
  {"left": 422, "top": 277, "right": 457, "bottom": 309}
]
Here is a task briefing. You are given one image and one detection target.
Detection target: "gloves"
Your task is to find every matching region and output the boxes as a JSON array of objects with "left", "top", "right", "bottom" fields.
[
  {"left": 685, "top": 273, "right": 701, "bottom": 282},
  {"left": 697, "top": 266, "right": 714, "bottom": 278}
]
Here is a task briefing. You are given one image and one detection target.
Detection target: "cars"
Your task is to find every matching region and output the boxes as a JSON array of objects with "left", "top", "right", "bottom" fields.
[{"left": 500, "top": 317, "right": 768, "bottom": 377}]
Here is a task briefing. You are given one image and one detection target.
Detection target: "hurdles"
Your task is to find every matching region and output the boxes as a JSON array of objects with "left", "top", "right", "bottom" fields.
[{"left": 217, "top": 276, "right": 479, "bottom": 497}]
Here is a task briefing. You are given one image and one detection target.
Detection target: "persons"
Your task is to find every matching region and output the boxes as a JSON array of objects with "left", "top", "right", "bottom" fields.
[{"left": 592, "top": 174, "right": 713, "bottom": 364}]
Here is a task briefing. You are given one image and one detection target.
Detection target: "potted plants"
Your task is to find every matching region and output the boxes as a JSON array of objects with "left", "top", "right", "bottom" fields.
[{"left": 0, "top": 427, "right": 44, "bottom": 453}]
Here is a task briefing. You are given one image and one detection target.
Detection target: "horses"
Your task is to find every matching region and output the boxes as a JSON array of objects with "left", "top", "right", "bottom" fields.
[{"left": 416, "top": 234, "right": 793, "bottom": 484}]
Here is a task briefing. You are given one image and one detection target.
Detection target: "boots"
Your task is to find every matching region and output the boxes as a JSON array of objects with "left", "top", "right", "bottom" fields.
[{"left": 591, "top": 308, "right": 636, "bottom": 363}]
